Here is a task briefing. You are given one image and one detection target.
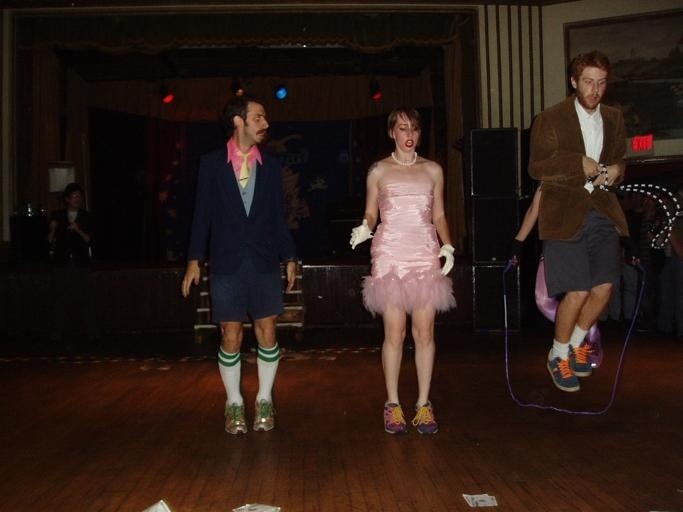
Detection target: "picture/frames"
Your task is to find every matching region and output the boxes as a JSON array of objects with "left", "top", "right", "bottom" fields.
[{"left": 562, "top": 8, "right": 683, "bottom": 140}]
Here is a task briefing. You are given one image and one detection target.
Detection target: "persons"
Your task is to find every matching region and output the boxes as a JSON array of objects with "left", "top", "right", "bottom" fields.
[
  {"left": 178, "top": 91, "right": 302, "bottom": 439},
  {"left": 49, "top": 182, "right": 103, "bottom": 252},
  {"left": 591, "top": 178, "right": 682, "bottom": 340},
  {"left": 502, "top": 177, "right": 641, "bottom": 371},
  {"left": 342, "top": 100, "right": 460, "bottom": 438},
  {"left": 522, "top": 48, "right": 626, "bottom": 396}
]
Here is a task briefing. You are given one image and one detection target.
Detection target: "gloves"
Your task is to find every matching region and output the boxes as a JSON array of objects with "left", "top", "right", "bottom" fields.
[
  {"left": 349, "top": 219, "right": 373, "bottom": 249},
  {"left": 438, "top": 244, "right": 456, "bottom": 278}
]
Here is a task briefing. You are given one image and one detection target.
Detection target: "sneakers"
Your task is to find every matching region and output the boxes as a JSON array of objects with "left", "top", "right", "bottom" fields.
[
  {"left": 585, "top": 327, "right": 604, "bottom": 370},
  {"left": 545, "top": 347, "right": 581, "bottom": 393},
  {"left": 568, "top": 341, "right": 593, "bottom": 378},
  {"left": 221, "top": 398, "right": 248, "bottom": 436},
  {"left": 382, "top": 400, "right": 406, "bottom": 435},
  {"left": 251, "top": 399, "right": 277, "bottom": 432},
  {"left": 414, "top": 399, "right": 440, "bottom": 435}
]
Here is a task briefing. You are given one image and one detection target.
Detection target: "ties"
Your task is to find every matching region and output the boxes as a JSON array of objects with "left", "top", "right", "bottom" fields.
[{"left": 236, "top": 150, "right": 251, "bottom": 189}]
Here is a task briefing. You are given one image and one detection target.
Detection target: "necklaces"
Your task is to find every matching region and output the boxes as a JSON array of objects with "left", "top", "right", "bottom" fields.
[{"left": 389, "top": 150, "right": 418, "bottom": 168}]
